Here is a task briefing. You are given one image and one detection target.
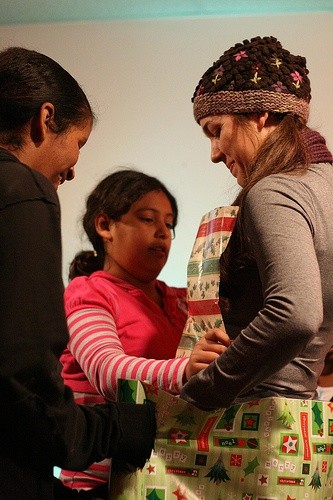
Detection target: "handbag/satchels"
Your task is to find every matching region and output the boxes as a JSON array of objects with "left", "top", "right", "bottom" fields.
[{"left": 110, "top": 379, "right": 333, "bottom": 500}]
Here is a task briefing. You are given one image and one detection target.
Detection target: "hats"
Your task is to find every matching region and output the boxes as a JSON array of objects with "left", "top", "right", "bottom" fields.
[{"left": 191, "top": 36, "right": 311, "bottom": 125}]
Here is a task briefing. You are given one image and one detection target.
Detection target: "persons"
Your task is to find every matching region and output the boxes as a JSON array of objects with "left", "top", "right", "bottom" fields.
[
  {"left": 0, "top": 47, "right": 157, "bottom": 500},
  {"left": 179, "top": 36, "right": 333, "bottom": 412},
  {"left": 59, "top": 169, "right": 231, "bottom": 495}
]
